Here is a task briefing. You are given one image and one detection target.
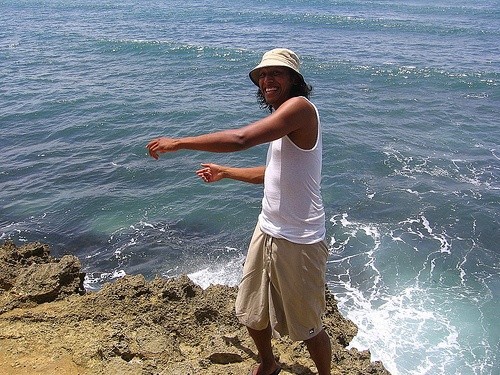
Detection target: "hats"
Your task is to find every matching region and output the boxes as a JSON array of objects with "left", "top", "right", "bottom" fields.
[{"left": 248, "top": 47, "right": 304, "bottom": 88}]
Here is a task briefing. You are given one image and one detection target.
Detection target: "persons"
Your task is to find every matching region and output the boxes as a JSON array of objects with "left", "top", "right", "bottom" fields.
[{"left": 145, "top": 46, "right": 332, "bottom": 374}]
